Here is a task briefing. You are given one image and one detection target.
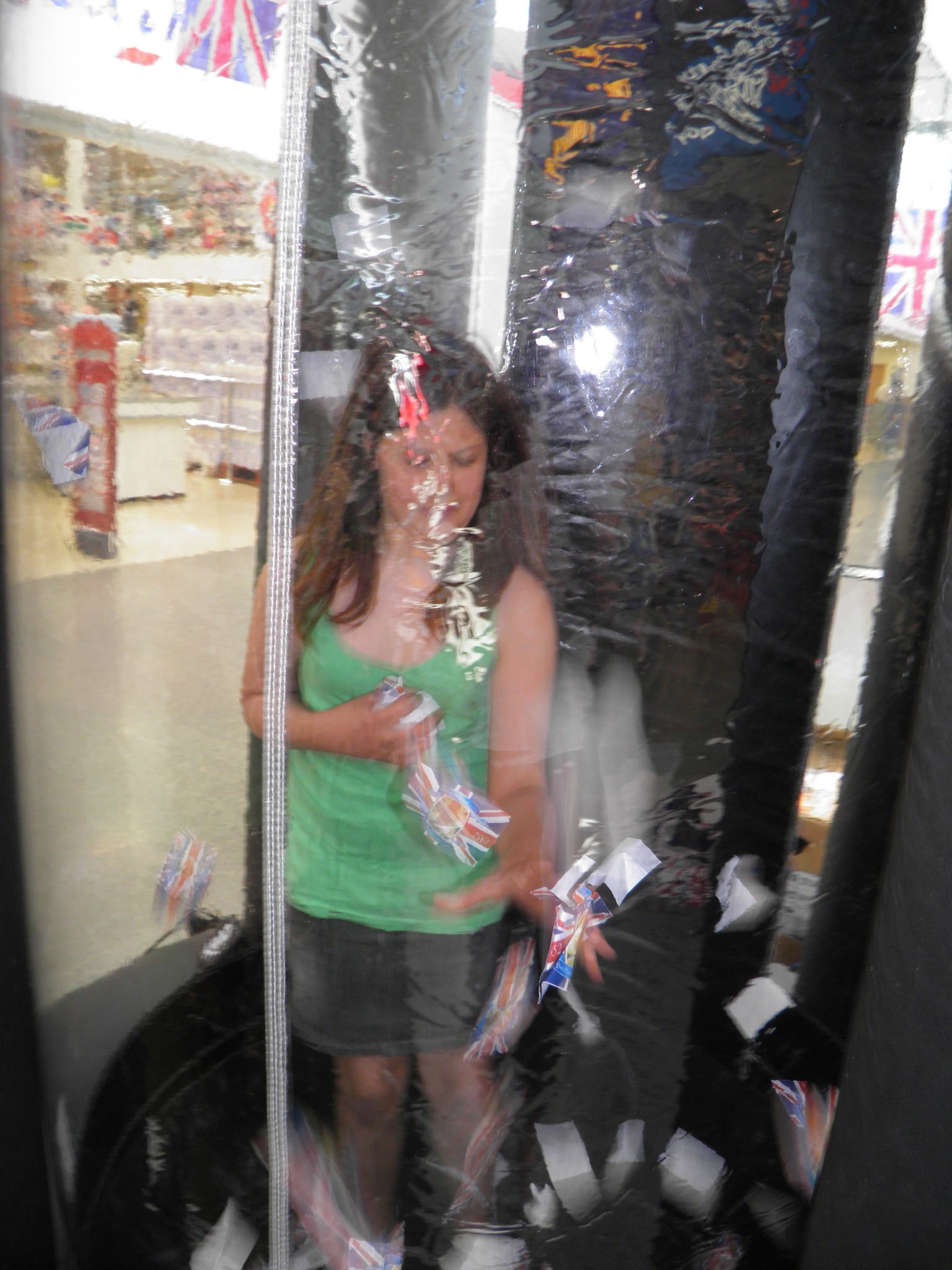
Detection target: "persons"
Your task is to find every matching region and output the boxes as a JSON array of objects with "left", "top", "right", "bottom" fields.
[
  {"left": 49, "top": 278, "right": 140, "bottom": 335},
  {"left": 238, "top": 328, "right": 609, "bottom": 1270}
]
